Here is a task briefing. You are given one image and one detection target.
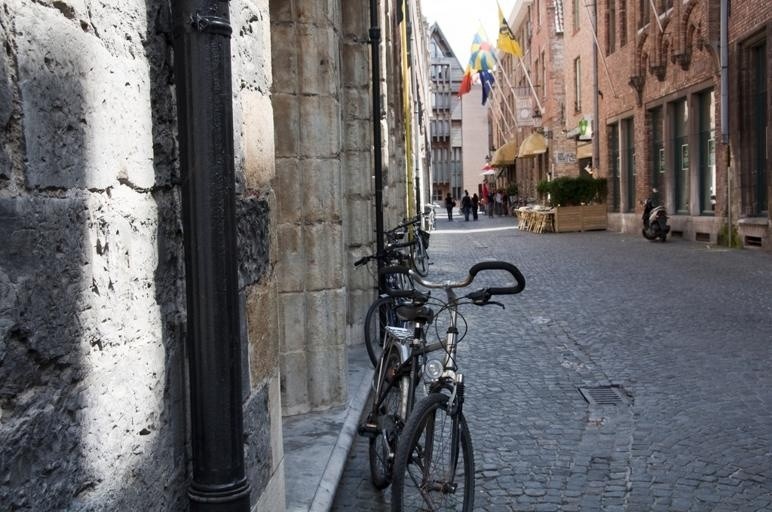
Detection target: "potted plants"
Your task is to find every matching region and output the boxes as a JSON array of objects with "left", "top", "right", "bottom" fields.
[
  {"left": 533, "top": 174, "right": 585, "bottom": 236},
  {"left": 580, "top": 171, "right": 609, "bottom": 233}
]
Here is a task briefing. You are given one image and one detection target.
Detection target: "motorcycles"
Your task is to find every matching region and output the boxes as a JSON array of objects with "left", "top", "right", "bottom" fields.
[{"left": 641, "top": 196, "right": 672, "bottom": 243}]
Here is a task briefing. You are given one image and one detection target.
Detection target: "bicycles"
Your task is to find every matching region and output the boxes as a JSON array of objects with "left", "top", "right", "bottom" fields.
[
  {"left": 374, "top": 217, "right": 420, "bottom": 305},
  {"left": 399, "top": 210, "right": 434, "bottom": 278},
  {"left": 356, "top": 259, "right": 527, "bottom": 510},
  {"left": 352, "top": 239, "right": 434, "bottom": 389}
]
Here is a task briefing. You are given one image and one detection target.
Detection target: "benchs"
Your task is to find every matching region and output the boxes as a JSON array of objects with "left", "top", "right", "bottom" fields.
[{"left": 512, "top": 205, "right": 556, "bottom": 235}]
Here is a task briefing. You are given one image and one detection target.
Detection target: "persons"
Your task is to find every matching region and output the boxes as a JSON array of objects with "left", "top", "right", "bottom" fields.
[
  {"left": 444, "top": 193, "right": 453, "bottom": 221},
  {"left": 461, "top": 190, "right": 509, "bottom": 221}
]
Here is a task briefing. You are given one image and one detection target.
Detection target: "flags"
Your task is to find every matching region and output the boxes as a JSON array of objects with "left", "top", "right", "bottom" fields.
[
  {"left": 457, "top": 63, "right": 478, "bottom": 97},
  {"left": 468, "top": 26, "right": 497, "bottom": 70},
  {"left": 478, "top": 70, "right": 496, "bottom": 105},
  {"left": 495, "top": 3, "right": 522, "bottom": 57}
]
